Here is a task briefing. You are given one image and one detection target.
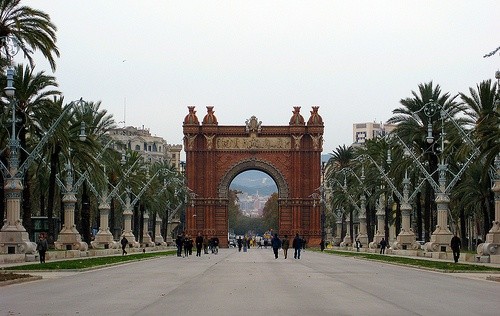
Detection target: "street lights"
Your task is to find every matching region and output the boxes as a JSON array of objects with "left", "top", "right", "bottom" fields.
[
  {"left": 308, "top": 133, "right": 438, "bottom": 248},
  {"left": 0, "top": 69, "right": 198, "bottom": 253},
  {"left": 413, "top": 98, "right": 480, "bottom": 252}
]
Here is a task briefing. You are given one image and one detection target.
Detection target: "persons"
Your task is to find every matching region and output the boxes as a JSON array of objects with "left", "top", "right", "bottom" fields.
[
  {"left": 233, "top": 233, "right": 268, "bottom": 251},
  {"left": 292, "top": 233, "right": 302, "bottom": 260},
  {"left": 356, "top": 238, "right": 361, "bottom": 251},
  {"left": 450, "top": 232, "right": 462, "bottom": 263},
  {"left": 320, "top": 239, "right": 325, "bottom": 252},
  {"left": 120, "top": 234, "right": 129, "bottom": 255},
  {"left": 36, "top": 232, "right": 49, "bottom": 264},
  {"left": 300, "top": 236, "right": 306, "bottom": 251},
  {"left": 281, "top": 234, "right": 290, "bottom": 259},
  {"left": 176, "top": 231, "right": 220, "bottom": 256},
  {"left": 271, "top": 234, "right": 281, "bottom": 260},
  {"left": 379, "top": 237, "right": 386, "bottom": 254}
]
